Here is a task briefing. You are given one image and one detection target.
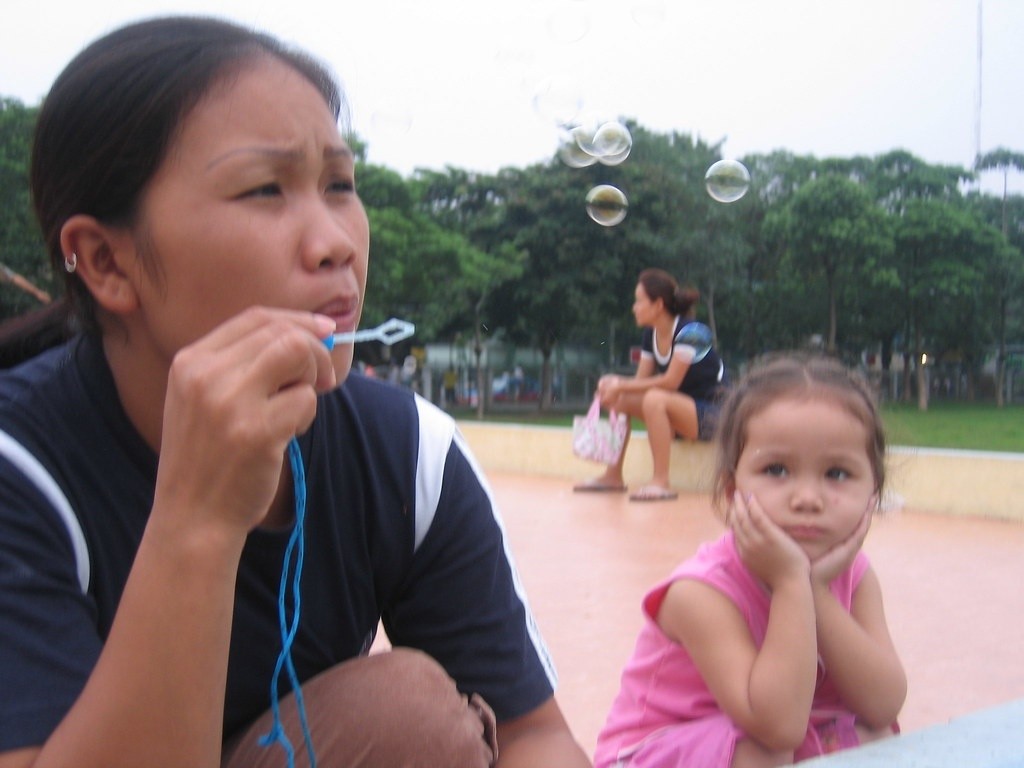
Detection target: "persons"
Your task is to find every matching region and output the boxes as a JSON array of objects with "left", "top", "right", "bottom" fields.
[
  {"left": 364, "top": 355, "right": 399, "bottom": 383},
  {"left": 592, "top": 359, "right": 908, "bottom": 768},
  {"left": 514, "top": 363, "right": 524, "bottom": 386},
  {"left": 573, "top": 268, "right": 730, "bottom": 502},
  {"left": 0, "top": 17, "right": 592, "bottom": 768},
  {"left": 444, "top": 367, "right": 458, "bottom": 401}
]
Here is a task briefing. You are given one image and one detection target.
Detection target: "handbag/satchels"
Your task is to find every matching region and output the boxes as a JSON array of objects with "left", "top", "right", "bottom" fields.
[{"left": 572, "top": 381, "right": 627, "bottom": 466}]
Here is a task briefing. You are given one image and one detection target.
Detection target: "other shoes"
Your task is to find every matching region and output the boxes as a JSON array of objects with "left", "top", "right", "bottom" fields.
[
  {"left": 629, "top": 485, "right": 678, "bottom": 499},
  {"left": 573, "top": 478, "right": 626, "bottom": 491}
]
